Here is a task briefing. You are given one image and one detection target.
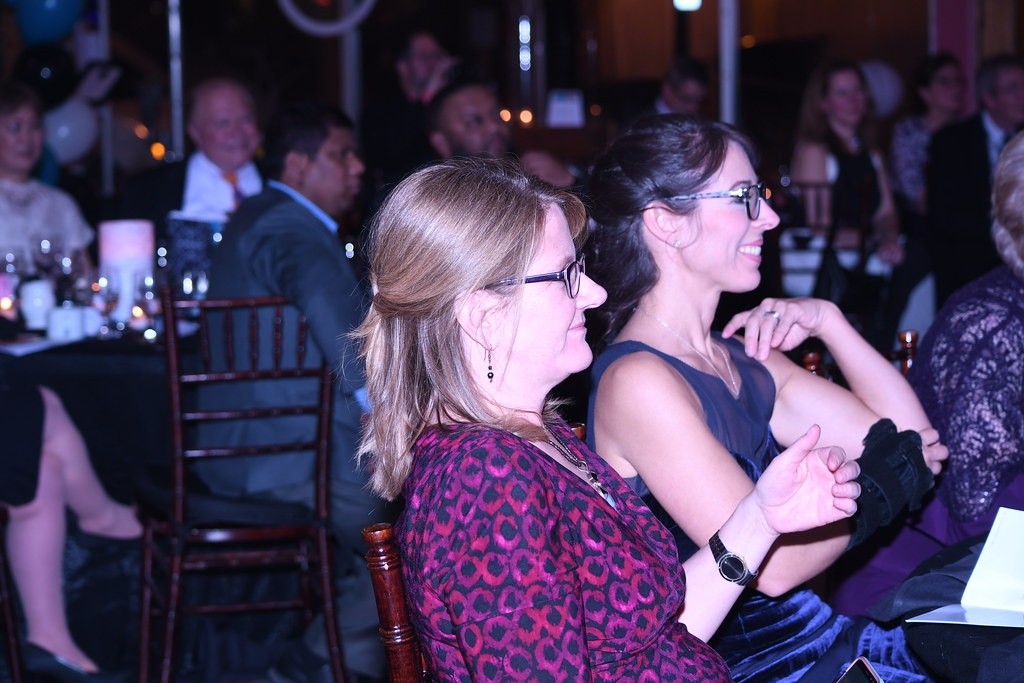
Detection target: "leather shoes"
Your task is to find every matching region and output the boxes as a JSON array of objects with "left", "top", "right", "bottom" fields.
[
  {"left": 76, "top": 499, "right": 172, "bottom": 551},
  {"left": 19, "top": 639, "right": 108, "bottom": 683}
]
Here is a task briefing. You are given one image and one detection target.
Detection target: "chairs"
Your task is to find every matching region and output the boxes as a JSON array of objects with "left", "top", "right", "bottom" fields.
[{"left": 0, "top": 181, "right": 919, "bottom": 683}]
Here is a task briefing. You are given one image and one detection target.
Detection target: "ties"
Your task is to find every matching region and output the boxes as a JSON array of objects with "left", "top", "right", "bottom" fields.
[{"left": 223, "top": 170, "right": 244, "bottom": 208}]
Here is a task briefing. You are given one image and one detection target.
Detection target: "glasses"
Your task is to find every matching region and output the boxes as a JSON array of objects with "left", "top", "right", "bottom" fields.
[
  {"left": 661, "top": 181, "right": 768, "bottom": 220},
  {"left": 478, "top": 252, "right": 586, "bottom": 298}
]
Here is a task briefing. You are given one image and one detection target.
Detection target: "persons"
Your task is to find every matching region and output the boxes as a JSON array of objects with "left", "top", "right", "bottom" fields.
[
  {"left": 0, "top": 350, "right": 144, "bottom": 683},
  {"left": 351, "top": 166, "right": 861, "bottom": 683},
  {"left": 141, "top": 80, "right": 280, "bottom": 219},
  {"left": 374, "top": 32, "right": 578, "bottom": 187},
  {"left": 575, "top": 114, "right": 949, "bottom": 683},
  {"left": 833, "top": 130, "right": 1024, "bottom": 620},
  {"left": 651, "top": 58, "right": 705, "bottom": 117},
  {"left": 196, "top": 105, "right": 392, "bottom": 683},
  {"left": 793, "top": 51, "right": 1024, "bottom": 293},
  {"left": 0, "top": 86, "right": 94, "bottom": 264}
]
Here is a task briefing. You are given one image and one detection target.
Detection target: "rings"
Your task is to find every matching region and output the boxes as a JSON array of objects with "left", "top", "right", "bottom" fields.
[{"left": 768, "top": 310, "right": 780, "bottom": 323}]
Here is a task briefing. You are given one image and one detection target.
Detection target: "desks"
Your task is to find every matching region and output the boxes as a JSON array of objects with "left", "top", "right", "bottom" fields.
[
  {"left": 773, "top": 223, "right": 935, "bottom": 378},
  {"left": 788, "top": 506, "right": 1024, "bottom": 683},
  {"left": 0, "top": 270, "right": 202, "bottom": 551}
]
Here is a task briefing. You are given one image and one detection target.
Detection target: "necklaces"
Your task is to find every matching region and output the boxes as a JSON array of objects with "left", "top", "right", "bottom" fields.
[
  {"left": 541, "top": 423, "right": 608, "bottom": 500},
  {"left": 633, "top": 308, "right": 739, "bottom": 396}
]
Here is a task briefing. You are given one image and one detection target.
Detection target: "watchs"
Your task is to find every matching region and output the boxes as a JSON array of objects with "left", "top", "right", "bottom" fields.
[{"left": 709, "top": 529, "right": 759, "bottom": 585}]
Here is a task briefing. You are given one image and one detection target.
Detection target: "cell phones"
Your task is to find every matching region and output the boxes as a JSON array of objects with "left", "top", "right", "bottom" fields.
[{"left": 836, "top": 656, "right": 883, "bottom": 683}]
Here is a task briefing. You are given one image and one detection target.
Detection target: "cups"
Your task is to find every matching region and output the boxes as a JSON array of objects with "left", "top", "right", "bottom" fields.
[{"left": 1, "top": 227, "right": 211, "bottom": 344}]
